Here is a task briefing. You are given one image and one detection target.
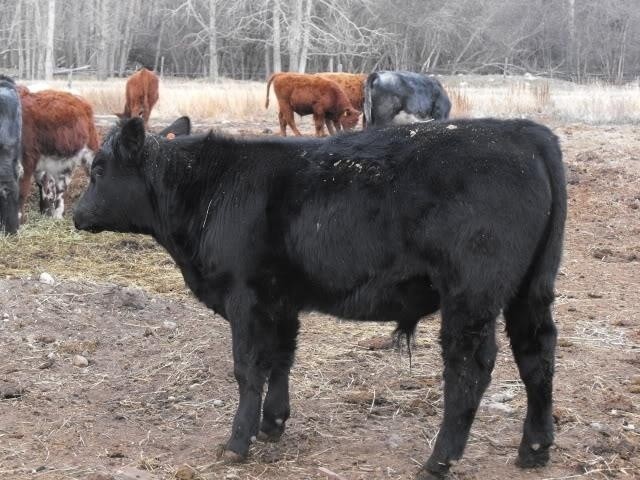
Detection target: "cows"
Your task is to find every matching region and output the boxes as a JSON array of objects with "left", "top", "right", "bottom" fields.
[
  {"left": 264, "top": 71, "right": 362, "bottom": 137},
  {"left": 363, "top": 69, "right": 451, "bottom": 129},
  {"left": 314, "top": 73, "right": 369, "bottom": 135},
  {"left": 0, "top": 74, "right": 101, "bottom": 238},
  {"left": 114, "top": 67, "right": 160, "bottom": 125},
  {"left": 73, "top": 116, "right": 567, "bottom": 480}
]
